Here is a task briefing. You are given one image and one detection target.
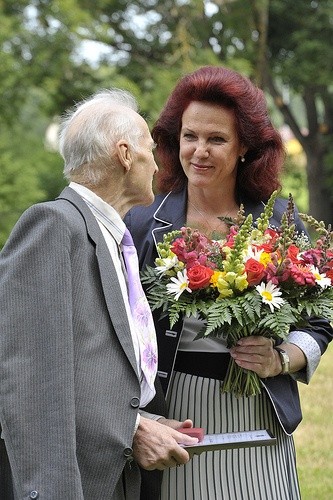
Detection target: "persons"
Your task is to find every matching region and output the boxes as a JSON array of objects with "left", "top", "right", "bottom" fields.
[
  {"left": 122, "top": 67, "right": 333, "bottom": 500},
  {"left": 0, "top": 84, "right": 199, "bottom": 500}
]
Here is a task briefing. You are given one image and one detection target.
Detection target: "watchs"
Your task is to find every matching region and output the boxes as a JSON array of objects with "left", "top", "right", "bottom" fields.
[{"left": 274, "top": 346, "right": 290, "bottom": 374}]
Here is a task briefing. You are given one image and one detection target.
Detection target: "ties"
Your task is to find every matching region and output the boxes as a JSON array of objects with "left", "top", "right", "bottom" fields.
[{"left": 120, "top": 227, "right": 158, "bottom": 391}]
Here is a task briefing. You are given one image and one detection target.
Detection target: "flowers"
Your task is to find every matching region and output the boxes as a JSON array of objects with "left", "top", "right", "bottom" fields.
[{"left": 136, "top": 188, "right": 333, "bottom": 399}]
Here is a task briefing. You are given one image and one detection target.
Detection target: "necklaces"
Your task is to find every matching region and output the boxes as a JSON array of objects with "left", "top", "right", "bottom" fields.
[{"left": 191, "top": 201, "right": 236, "bottom": 231}]
